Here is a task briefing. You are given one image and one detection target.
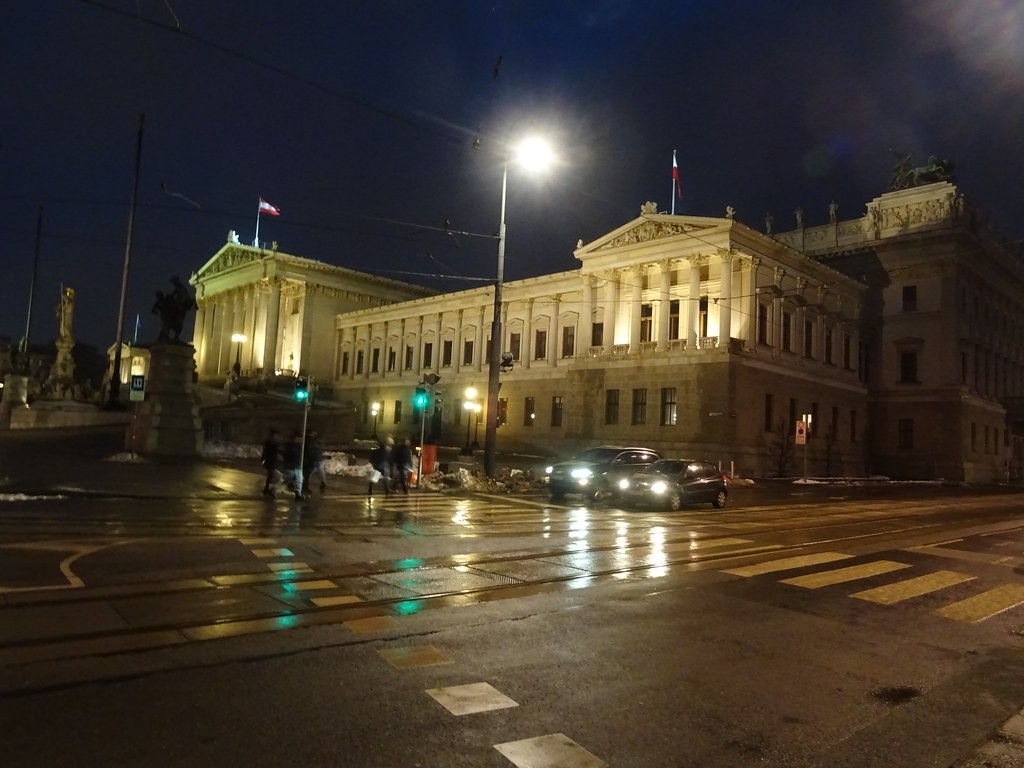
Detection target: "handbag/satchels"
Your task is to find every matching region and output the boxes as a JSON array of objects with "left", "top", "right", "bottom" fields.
[
  {"left": 407, "top": 470, "right": 418, "bottom": 488},
  {"left": 369, "top": 445, "right": 383, "bottom": 464},
  {"left": 270, "top": 469, "right": 284, "bottom": 488},
  {"left": 365, "top": 467, "right": 381, "bottom": 483}
]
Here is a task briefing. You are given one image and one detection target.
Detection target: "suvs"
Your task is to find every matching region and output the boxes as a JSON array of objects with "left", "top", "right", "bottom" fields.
[{"left": 546, "top": 444, "right": 667, "bottom": 505}]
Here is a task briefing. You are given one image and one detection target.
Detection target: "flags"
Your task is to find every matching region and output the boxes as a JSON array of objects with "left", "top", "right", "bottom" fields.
[
  {"left": 258, "top": 196, "right": 281, "bottom": 216},
  {"left": 672, "top": 152, "right": 685, "bottom": 200}
]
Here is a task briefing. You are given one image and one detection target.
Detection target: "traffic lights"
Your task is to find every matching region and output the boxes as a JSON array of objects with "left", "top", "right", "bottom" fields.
[
  {"left": 415, "top": 386, "right": 427, "bottom": 408},
  {"left": 294, "top": 380, "right": 307, "bottom": 401},
  {"left": 429, "top": 373, "right": 440, "bottom": 385}
]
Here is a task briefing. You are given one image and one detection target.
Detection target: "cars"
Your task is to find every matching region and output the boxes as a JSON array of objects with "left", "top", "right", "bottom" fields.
[{"left": 619, "top": 458, "right": 729, "bottom": 511}]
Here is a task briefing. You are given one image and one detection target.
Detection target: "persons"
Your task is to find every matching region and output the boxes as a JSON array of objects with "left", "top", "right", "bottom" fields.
[
  {"left": 956, "top": 193, "right": 968, "bottom": 216},
  {"left": 261, "top": 427, "right": 327, "bottom": 500},
  {"left": 367, "top": 437, "right": 413, "bottom": 497},
  {"left": 794, "top": 205, "right": 804, "bottom": 229},
  {"left": 828, "top": 200, "right": 838, "bottom": 221},
  {"left": 765, "top": 212, "right": 774, "bottom": 236},
  {"left": 151, "top": 286, "right": 184, "bottom": 345}
]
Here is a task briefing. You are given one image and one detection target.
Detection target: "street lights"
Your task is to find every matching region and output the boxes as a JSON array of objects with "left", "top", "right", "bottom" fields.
[
  {"left": 232, "top": 333, "right": 247, "bottom": 375},
  {"left": 474, "top": 405, "right": 482, "bottom": 449},
  {"left": 372, "top": 403, "right": 380, "bottom": 439},
  {"left": 464, "top": 401, "right": 473, "bottom": 452},
  {"left": 482, "top": 135, "right": 547, "bottom": 480}
]
